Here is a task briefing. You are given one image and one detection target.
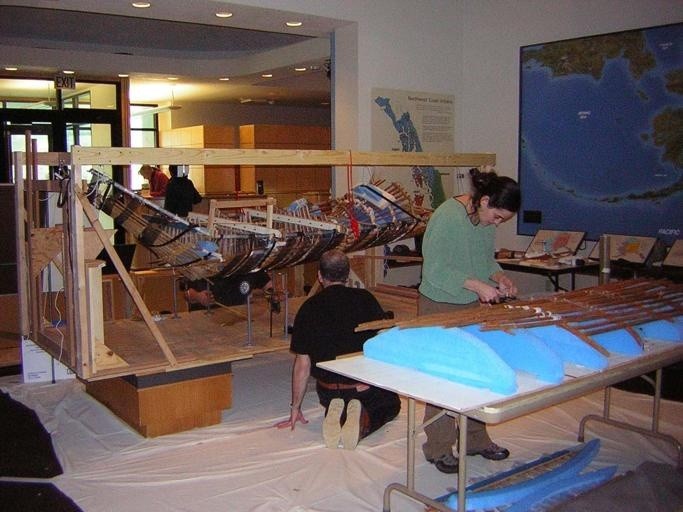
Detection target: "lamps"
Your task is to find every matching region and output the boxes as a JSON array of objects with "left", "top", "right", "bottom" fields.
[{"left": 130, "top": 83, "right": 183, "bottom": 118}]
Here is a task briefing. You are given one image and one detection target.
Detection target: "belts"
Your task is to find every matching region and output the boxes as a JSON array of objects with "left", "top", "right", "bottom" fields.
[{"left": 317, "top": 377, "right": 368, "bottom": 391}]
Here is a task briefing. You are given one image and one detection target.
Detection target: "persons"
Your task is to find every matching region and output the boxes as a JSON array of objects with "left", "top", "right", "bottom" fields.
[
  {"left": 177, "top": 268, "right": 292, "bottom": 313},
  {"left": 274, "top": 248, "right": 402, "bottom": 451},
  {"left": 417, "top": 167, "right": 522, "bottom": 474},
  {"left": 138, "top": 164, "right": 170, "bottom": 198},
  {"left": 163, "top": 164, "right": 203, "bottom": 218}
]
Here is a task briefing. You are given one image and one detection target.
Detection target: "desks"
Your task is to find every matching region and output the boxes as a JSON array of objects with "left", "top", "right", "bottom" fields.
[
  {"left": 313, "top": 282, "right": 683, "bottom": 512},
  {"left": 488, "top": 252, "right": 600, "bottom": 292}
]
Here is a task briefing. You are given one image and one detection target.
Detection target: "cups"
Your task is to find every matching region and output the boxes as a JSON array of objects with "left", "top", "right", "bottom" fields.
[{"left": 598, "top": 235, "right": 611, "bottom": 274}]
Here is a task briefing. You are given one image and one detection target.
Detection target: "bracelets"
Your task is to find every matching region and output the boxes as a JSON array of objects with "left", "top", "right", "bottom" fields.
[{"left": 287, "top": 401, "right": 301, "bottom": 411}]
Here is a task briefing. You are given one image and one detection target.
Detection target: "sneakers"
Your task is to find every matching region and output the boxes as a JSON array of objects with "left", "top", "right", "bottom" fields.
[
  {"left": 457, "top": 444, "right": 511, "bottom": 461},
  {"left": 340, "top": 398, "right": 368, "bottom": 450},
  {"left": 427, "top": 454, "right": 459, "bottom": 474},
  {"left": 322, "top": 397, "right": 346, "bottom": 448}
]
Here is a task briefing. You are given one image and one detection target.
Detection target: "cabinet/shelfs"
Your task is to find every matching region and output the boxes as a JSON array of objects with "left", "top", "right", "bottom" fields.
[{"left": 170, "top": 123, "right": 331, "bottom": 218}]
